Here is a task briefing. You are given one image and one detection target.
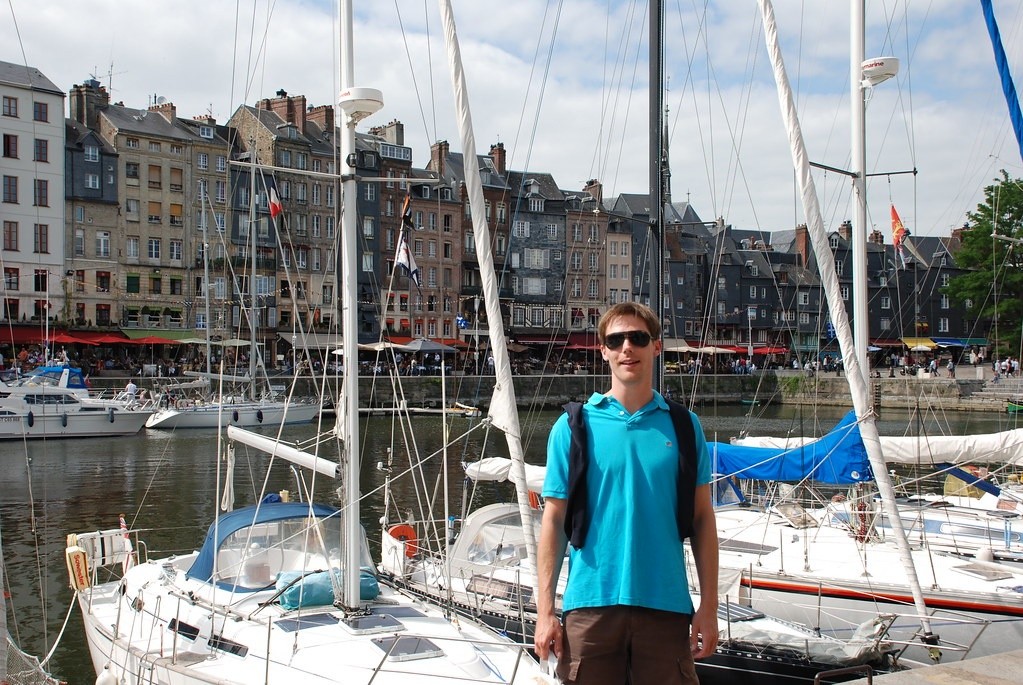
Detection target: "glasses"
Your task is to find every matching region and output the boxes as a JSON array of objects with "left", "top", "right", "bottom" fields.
[{"left": 604, "top": 330, "right": 654, "bottom": 350}]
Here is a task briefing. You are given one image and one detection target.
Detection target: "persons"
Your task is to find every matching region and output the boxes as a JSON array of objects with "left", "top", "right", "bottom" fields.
[
  {"left": 533, "top": 298, "right": 720, "bottom": 684},
  {"left": 665, "top": 346, "right": 1020, "bottom": 383},
  {"left": 0, "top": 341, "right": 608, "bottom": 374}
]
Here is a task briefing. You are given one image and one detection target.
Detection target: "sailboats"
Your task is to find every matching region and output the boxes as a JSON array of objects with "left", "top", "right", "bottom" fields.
[{"left": 0, "top": 1, "right": 1023, "bottom": 685}]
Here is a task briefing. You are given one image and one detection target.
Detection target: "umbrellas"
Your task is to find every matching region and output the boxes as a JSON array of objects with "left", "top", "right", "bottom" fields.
[
  {"left": 688, "top": 344, "right": 787, "bottom": 361},
  {"left": 45, "top": 333, "right": 265, "bottom": 376},
  {"left": 357, "top": 338, "right": 459, "bottom": 374}
]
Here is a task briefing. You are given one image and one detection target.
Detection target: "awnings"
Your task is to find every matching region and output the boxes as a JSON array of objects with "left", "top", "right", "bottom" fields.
[
  {"left": 870, "top": 338, "right": 905, "bottom": 346},
  {"left": 275, "top": 331, "right": 346, "bottom": 348},
  {"left": 958, "top": 337, "right": 991, "bottom": 345},
  {"left": 664, "top": 337, "right": 690, "bottom": 352},
  {"left": 385, "top": 335, "right": 469, "bottom": 347},
  {"left": 900, "top": 335, "right": 937, "bottom": 348},
  {"left": 932, "top": 336, "right": 969, "bottom": 347}
]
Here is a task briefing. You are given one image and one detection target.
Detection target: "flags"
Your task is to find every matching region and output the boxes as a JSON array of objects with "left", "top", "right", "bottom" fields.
[
  {"left": 270, "top": 188, "right": 282, "bottom": 219},
  {"left": 892, "top": 204, "right": 908, "bottom": 249},
  {"left": 394, "top": 195, "right": 422, "bottom": 294}
]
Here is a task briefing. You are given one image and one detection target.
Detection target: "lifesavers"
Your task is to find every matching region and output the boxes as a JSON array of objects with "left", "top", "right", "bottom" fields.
[{"left": 387, "top": 526, "right": 418, "bottom": 557}]
